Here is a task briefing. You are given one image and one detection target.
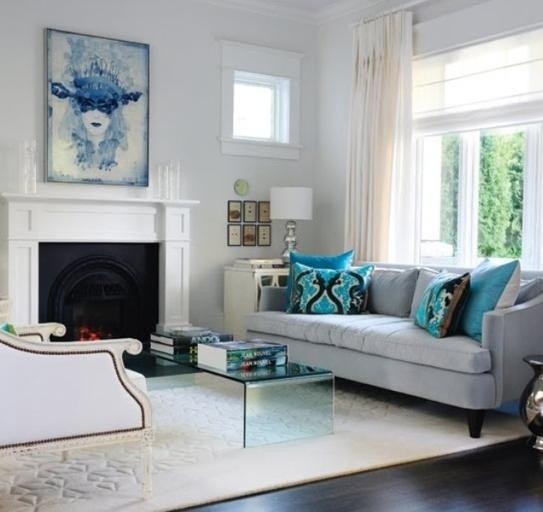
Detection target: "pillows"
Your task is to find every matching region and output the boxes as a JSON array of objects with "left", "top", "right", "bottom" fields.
[
  {"left": 287, "top": 247, "right": 355, "bottom": 313},
  {"left": 284, "top": 260, "right": 376, "bottom": 313},
  {"left": 413, "top": 267, "right": 471, "bottom": 340},
  {"left": 460, "top": 257, "right": 520, "bottom": 343}
]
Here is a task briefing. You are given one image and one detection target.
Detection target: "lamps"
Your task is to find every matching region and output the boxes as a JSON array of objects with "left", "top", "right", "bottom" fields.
[{"left": 270, "top": 187, "right": 312, "bottom": 262}]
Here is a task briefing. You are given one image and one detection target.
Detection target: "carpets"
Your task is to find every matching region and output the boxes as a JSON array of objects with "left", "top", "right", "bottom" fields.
[{"left": 0, "top": 371, "right": 543, "bottom": 512}]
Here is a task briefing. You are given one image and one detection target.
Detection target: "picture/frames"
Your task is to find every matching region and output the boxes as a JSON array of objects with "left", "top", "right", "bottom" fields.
[
  {"left": 234, "top": 179, "right": 250, "bottom": 194},
  {"left": 244, "top": 200, "right": 258, "bottom": 222},
  {"left": 258, "top": 225, "right": 271, "bottom": 246},
  {"left": 227, "top": 224, "right": 241, "bottom": 245},
  {"left": 259, "top": 202, "right": 270, "bottom": 223},
  {"left": 243, "top": 224, "right": 256, "bottom": 246},
  {"left": 228, "top": 202, "right": 243, "bottom": 222},
  {"left": 43, "top": 26, "right": 150, "bottom": 188}
]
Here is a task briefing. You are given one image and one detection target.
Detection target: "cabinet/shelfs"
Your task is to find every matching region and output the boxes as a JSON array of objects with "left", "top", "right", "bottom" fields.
[{"left": 224, "top": 267, "right": 290, "bottom": 337}]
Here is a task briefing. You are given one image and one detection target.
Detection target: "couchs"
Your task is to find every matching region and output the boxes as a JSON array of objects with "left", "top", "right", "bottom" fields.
[
  {"left": 0, "top": 321, "right": 150, "bottom": 449},
  {"left": 240, "top": 264, "right": 543, "bottom": 439}
]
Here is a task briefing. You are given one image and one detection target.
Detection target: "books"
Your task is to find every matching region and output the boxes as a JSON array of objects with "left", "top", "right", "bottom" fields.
[
  {"left": 197, "top": 337, "right": 289, "bottom": 374},
  {"left": 149, "top": 326, "right": 235, "bottom": 361},
  {"left": 234, "top": 255, "right": 284, "bottom": 269}
]
